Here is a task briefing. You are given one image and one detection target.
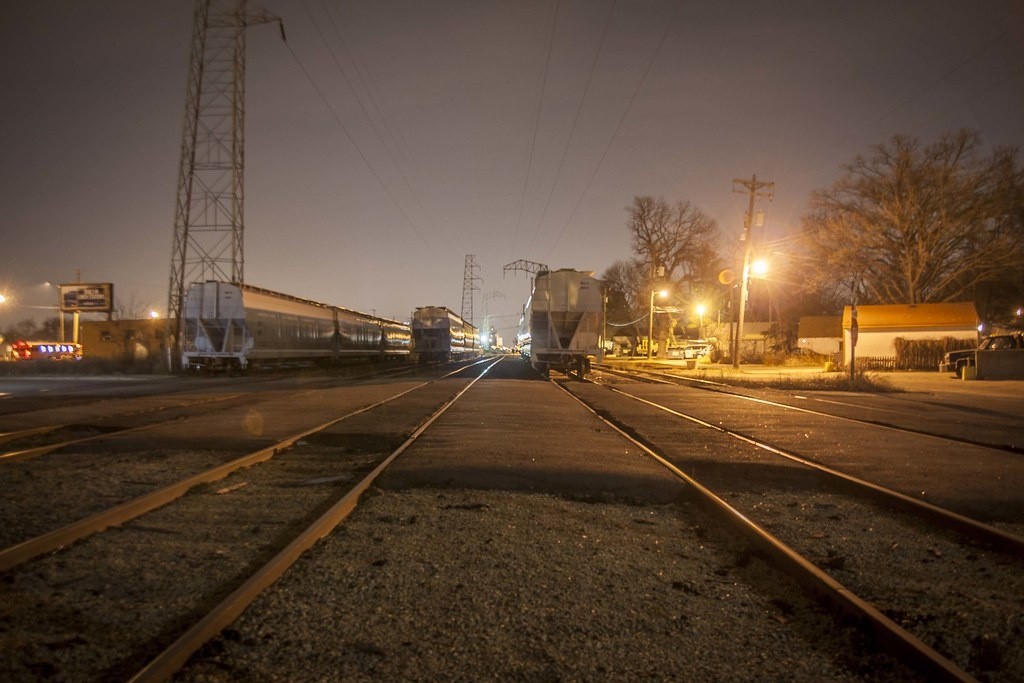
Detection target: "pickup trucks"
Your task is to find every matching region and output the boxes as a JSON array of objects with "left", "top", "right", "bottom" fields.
[{"left": 944, "top": 333, "right": 1024, "bottom": 379}]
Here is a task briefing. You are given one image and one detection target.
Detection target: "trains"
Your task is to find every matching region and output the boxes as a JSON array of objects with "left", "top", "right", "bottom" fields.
[
  {"left": 181, "top": 280, "right": 411, "bottom": 375},
  {"left": 409, "top": 305, "right": 486, "bottom": 363},
  {"left": 518, "top": 269, "right": 608, "bottom": 379}
]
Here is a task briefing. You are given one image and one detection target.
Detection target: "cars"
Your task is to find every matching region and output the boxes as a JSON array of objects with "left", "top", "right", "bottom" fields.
[{"left": 684, "top": 345, "right": 714, "bottom": 359}]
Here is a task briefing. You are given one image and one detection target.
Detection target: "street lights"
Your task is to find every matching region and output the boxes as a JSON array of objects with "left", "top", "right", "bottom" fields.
[
  {"left": 734, "top": 260, "right": 769, "bottom": 368},
  {"left": 647, "top": 288, "right": 670, "bottom": 359}
]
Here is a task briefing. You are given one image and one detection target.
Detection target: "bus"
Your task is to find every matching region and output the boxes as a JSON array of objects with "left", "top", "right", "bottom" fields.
[{"left": 8, "top": 341, "right": 84, "bottom": 362}]
